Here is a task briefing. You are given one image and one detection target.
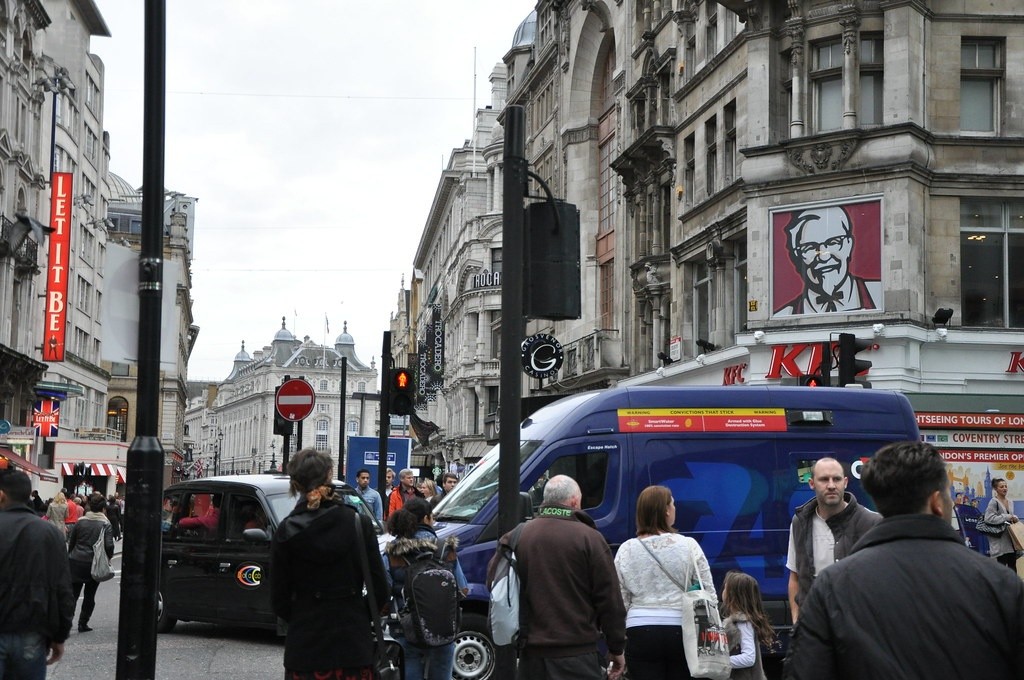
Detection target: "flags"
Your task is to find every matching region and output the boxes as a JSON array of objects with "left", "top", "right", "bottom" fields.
[
  {"left": 192, "top": 459, "right": 202, "bottom": 473},
  {"left": 34, "top": 401, "right": 60, "bottom": 437}
]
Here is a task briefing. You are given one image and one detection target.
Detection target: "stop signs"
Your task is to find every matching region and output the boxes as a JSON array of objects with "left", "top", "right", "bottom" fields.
[{"left": 276, "top": 379, "right": 316, "bottom": 422}]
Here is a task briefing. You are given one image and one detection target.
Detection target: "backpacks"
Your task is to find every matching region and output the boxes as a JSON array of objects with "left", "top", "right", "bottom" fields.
[
  {"left": 399, "top": 537, "right": 460, "bottom": 647},
  {"left": 487, "top": 523, "right": 525, "bottom": 646}
]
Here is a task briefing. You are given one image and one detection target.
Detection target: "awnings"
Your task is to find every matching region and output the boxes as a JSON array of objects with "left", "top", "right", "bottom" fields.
[
  {"left": 117, "top": 468, "right": 126, "bottom": 483},
  {"left": 0, "top": 450, "right": 58, "bottom": 483},
  {"left": 62, "top": 463, "right": 116, "bottom": 476}
]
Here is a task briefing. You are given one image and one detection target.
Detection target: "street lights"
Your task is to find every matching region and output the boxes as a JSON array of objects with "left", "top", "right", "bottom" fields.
[{"left": 217, "top": 432, "right": 223, "bottom": 474}]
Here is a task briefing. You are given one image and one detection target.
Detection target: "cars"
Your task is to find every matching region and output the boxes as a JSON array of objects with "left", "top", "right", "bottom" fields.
[{"left": 159, "top": 472, "right": 386, "bottom": 636}]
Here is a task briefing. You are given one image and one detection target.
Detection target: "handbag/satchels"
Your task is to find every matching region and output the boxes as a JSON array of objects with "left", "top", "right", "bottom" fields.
[
  {"left": 975, "top": 501, "right": 1007, "bottom": 538},
  {"left": 1006, "top": 519, "right": 1024, "bottom": 550},
  {"left": 680, "top": 536, "right": 733, "bottom": 679},
  {"left": 91, "top": 525, "right": 115, "bottom": 582},
  {"left": 371, "top": 632, "right": 404, "bottom": 680}
]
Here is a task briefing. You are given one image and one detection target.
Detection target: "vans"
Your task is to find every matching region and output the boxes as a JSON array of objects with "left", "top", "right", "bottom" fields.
[{"left": 380, "top": 386, "right": 924, "bottom": 680}]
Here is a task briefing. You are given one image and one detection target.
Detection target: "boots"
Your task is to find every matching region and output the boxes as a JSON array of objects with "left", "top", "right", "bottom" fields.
[{"left": 78, "top": 601, "right": 95, "bottom": 633}]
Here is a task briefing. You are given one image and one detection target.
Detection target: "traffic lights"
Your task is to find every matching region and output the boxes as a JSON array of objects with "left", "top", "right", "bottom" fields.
[
  {"left": 838, "top": 334, "right": 873, "bottom": 391},
  {"left": 800, "top": 375, "right": 823, "bottom": 386},
  {"left": 387, "top": 369, "right": 416, "bottom": 414}
]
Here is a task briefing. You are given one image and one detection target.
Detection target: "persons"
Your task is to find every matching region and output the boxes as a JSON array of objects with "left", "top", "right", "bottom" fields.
[
  {"left": 614, "top": 486, "right": 718, "bottom": 680},
  {"left": 984, "top": 478, "right": 1019, "bottom": 574},
  {"left": 783, "top": 443, "right": 1024, "bottom": 680},
  {"left": 68, "top": 497, "right": 114, "bottom": 632},
  {"left": 32, "top": 488, "right": 125, "bottom": 542},
  {"left": 786, "top": 458, "right": 882, "bottom": 625},
  {"left": 722, "top": 573, "right": 778, "bottom": 680},
  {"left": 382, "top": 498, "right": 469, "bottom": 680},
  {"left": 270, "top": 449, "right": 390, "bottom": 680},
  {"left": 487, "top": 475, "right": 626, "bottom": 680},
  {"left": 345, "top": 470, "right": 382, "bottom": 520},
  {"left": 385, "top": 468, "right": 457, "bottom": 516},
  {"left": 163, "top": 493, "right": 266, "bottom": 531},
  {"left": 0, "top": 470, "right": 73, "bottom": 680}
]
